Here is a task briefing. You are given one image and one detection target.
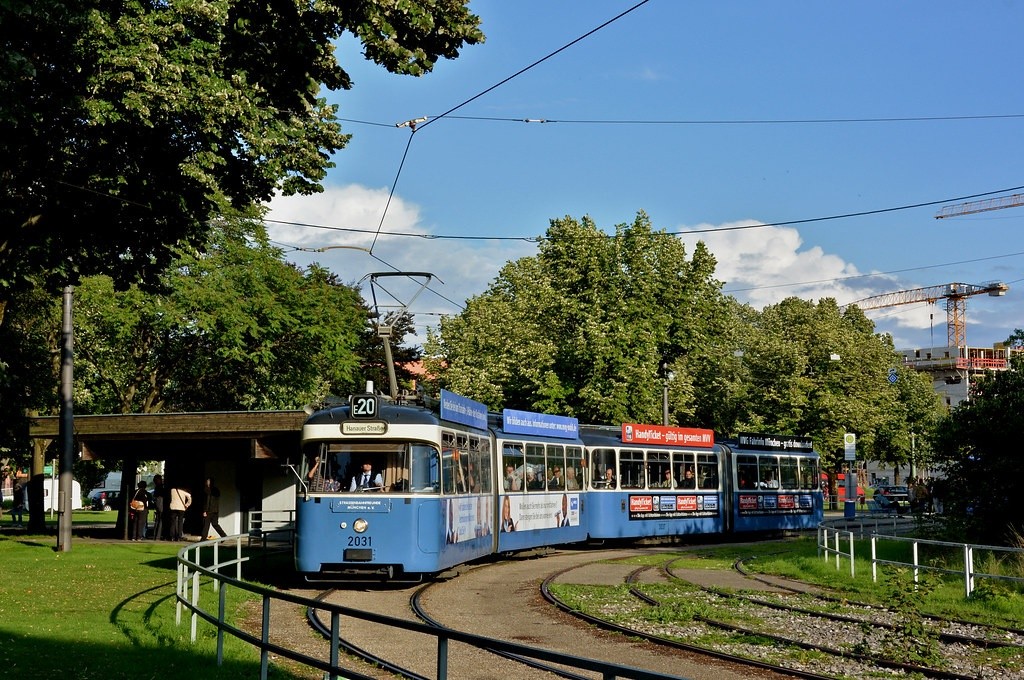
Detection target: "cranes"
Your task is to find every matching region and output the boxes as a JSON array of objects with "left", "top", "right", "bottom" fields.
[
  {"left": 837, "top": 281, "right": 1009, "bottom": 356},
  {"left": 934, "top": 193, "right": 1024, "bottom": 219}
]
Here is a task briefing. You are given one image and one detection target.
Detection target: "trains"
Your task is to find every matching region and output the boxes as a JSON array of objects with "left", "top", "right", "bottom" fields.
[{"left": 282, "top": 273, "right": 824, "bottom": 589}]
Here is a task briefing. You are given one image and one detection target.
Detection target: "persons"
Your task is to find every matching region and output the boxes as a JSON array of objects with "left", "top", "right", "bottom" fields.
[
  {"left": 661, "top": 470, "right": 678, "bottom": 489},
  {"left": 555, "top": 494, "right": 570, "bottom": 527},
  {"left": 130, "top": 474, "right": 192, "bottom": 541},
  {"left": 602, "top": 468, "right": 616, "bottom": 489},
  {"left": 500, "top": 496, "right": 515, "bottom": 532},
  {"left": 11, "top": 477, "right": 26, "bottom": 528},
  {"left": 349, "top": 459, "right": 384, "bottom": 492},
  {"left": 198, "top": 476, "right": 225, "bottom": 541},
  {"left": 677, "top": 471, "right": 695, "bottom": 489},
  {"left": 875, "top": 488, "right": 909, "bottom": 518},
  {"left": 502, "top": 465, "right": 580, "bottom": 492},
  {"left": 308, "top": 453, "right": 326, "bottom": 492}
]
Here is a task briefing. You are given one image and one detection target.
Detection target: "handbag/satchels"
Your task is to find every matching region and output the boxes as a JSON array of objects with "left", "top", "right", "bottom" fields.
[{"left": 130, "top": 499, "right": 145, "bottom": 511}]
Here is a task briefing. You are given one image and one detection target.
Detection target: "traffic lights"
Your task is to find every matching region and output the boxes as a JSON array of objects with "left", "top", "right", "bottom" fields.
[{"left": 21, "top": 467, "right": 29, "bottom": 474}]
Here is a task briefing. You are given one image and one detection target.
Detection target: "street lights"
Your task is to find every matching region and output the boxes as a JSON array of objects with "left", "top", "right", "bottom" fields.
[{"left": 663, "top": 363, "right": 676, "bottom": 427}]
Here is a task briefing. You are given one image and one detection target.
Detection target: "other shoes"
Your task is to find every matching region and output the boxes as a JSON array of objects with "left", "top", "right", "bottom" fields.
[{"left": 132, "top": 538, "right": 142, "bottom": 542}]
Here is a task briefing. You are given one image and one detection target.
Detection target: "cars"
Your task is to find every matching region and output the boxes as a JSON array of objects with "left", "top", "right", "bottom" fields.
[{"left": 145, "top": 489, "right": 157, "bottom": 509}]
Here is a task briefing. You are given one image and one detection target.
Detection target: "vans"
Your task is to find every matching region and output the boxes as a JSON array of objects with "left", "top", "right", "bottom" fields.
[
  {"left": 873, "top": 485, "right": 908, "bottom": 501},
  {"left": 87, "top": 488, "right": 122, "bottom": 511},
  {"left": 815, "top": 473, "right": 865, "bottom": 504}
]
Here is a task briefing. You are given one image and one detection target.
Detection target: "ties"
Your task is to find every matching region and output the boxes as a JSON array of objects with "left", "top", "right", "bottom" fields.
[{"left": 363, "top": 476, "right": 367, "bottom": 491}]
